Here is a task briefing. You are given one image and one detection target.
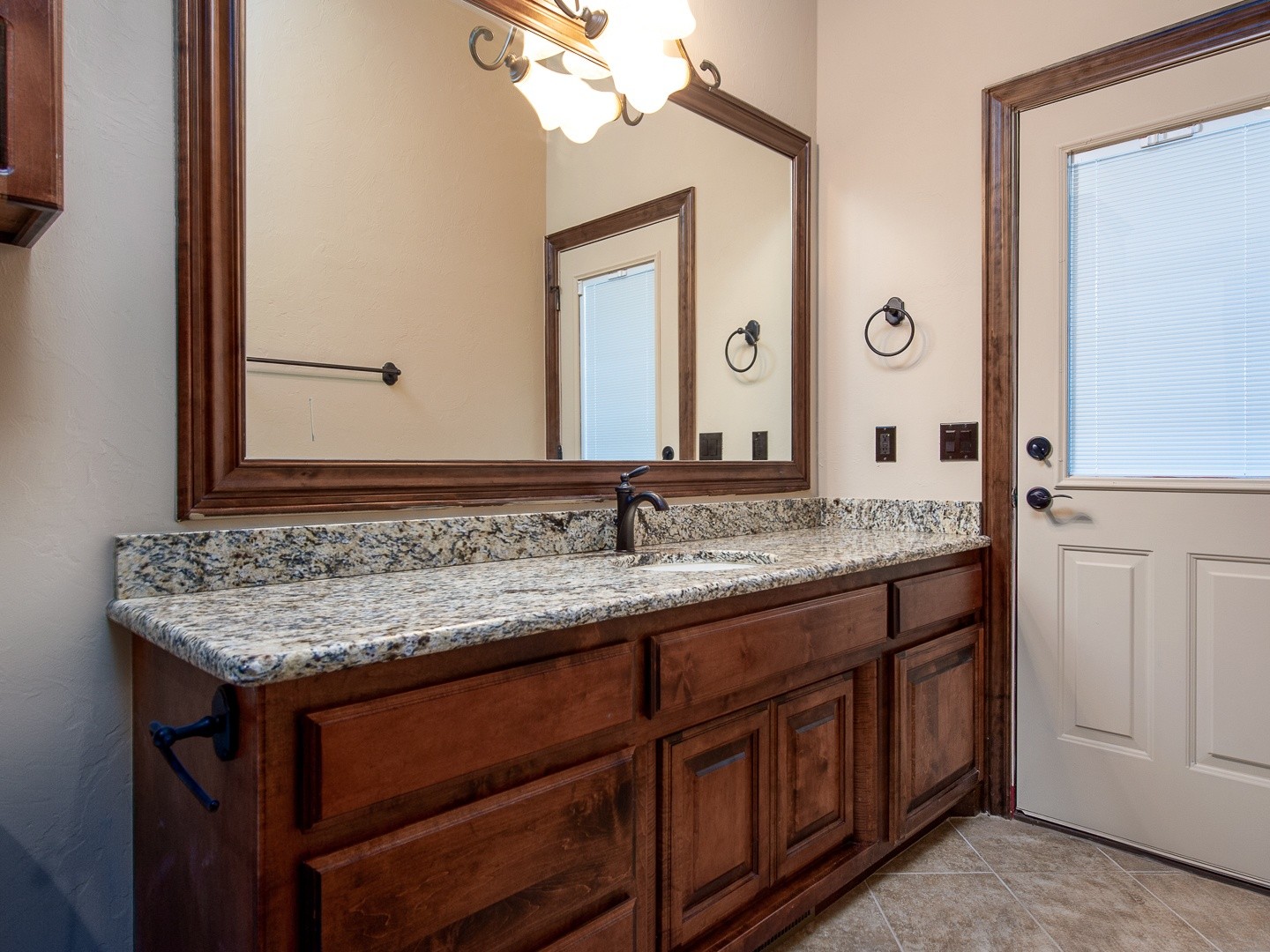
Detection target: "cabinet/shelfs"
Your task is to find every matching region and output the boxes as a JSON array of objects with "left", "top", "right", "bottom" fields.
[
  {"left": 133, "top": 548, "right": 995, "bottom": 952},
  {"left": 0, "top": 0, "right": 64, "bottom": 250}
]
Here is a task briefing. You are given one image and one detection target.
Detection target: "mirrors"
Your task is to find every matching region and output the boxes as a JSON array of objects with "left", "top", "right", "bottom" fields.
[{"left": 172, "top": 0, "right": 814, "bottom": 522}]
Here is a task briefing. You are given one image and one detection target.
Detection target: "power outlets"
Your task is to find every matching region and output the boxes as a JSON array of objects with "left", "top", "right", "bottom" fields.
[
  {"left": 875, "top": 426, "right": 897, "bottom": 461},
  {"left": 752, "top": 431, "right": 767, "bottom": 459}
]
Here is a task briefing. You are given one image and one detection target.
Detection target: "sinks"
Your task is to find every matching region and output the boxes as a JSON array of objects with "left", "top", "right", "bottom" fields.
[{"left": 612, "top": 549, "right": 780, "bottom": 573}]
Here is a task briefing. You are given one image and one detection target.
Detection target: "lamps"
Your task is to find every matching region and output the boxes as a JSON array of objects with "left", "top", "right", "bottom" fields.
[
  {"left": 554, "top": 0, "right": 697, "bottom": 114},
  {"left": 470, "top": 22, "right": 644, "bottom": 144}
]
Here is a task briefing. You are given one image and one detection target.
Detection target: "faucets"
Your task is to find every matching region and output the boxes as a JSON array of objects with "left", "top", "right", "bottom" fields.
[{"left": 613, "top": 465, "right": 671, "bottom": 553}]
